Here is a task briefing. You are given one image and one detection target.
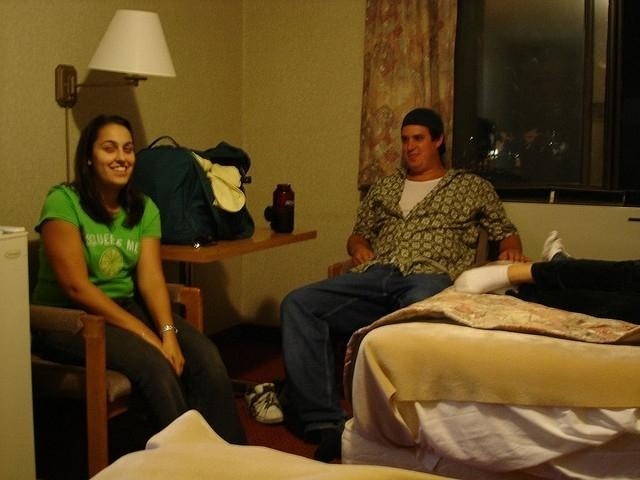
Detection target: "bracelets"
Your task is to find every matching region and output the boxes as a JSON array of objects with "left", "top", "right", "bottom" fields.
[{"left": 160, "top": 324, "right": 179, "bottom": 332}]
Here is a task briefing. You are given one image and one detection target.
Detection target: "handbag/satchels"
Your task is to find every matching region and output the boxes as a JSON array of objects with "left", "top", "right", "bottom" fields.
[{"left": 131, "top": 135, "right": 256, "bottom": 247}]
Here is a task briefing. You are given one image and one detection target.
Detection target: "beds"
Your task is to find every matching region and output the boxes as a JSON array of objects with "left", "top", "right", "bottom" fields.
[
  {"left": 339, "top": 254, "right": 640, "bottom": 480},
  {"left": 89, "top": 407, "right": 457, "bottom": 480}
]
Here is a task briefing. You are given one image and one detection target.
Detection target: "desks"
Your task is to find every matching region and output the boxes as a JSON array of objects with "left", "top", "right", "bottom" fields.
[
  {"left": 0, "top": 231, "right": 37, "bottom": 480},
  {"left": 160, "top": 224, "right": 318, "bottom": 318}
]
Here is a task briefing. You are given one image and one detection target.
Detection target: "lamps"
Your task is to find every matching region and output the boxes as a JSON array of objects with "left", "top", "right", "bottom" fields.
[{"left": 55, "top": 9, "right": 177, "bottom": 107}]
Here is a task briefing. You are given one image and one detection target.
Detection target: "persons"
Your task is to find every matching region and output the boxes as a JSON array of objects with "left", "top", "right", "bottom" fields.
[
  {"left": 30, "top": 113, "right": 250, "bottom": 447},
  {"left": 277, "top": 107, "right": 533, "bottom": 464},
  {"left": 454, "top": 230, "right": 640, "bottom": 327}
]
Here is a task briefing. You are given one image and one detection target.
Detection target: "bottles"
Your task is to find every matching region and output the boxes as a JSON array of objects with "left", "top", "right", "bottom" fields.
[{"left": 270, "top": 182, "right": 295, "bottom": 234}]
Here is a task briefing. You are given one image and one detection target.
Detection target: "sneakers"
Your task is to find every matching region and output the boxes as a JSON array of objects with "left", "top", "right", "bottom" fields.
[{"left": 244, "top": 381, "right": 285, "bottom": 426}]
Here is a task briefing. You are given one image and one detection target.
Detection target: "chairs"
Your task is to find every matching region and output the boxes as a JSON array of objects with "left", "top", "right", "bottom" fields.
[
  {"left": 327, "top": 230, "right": 489, "bottom": 405},
  {"left": 29, "top": 282, "right": 204, "bottom": 478}
]
